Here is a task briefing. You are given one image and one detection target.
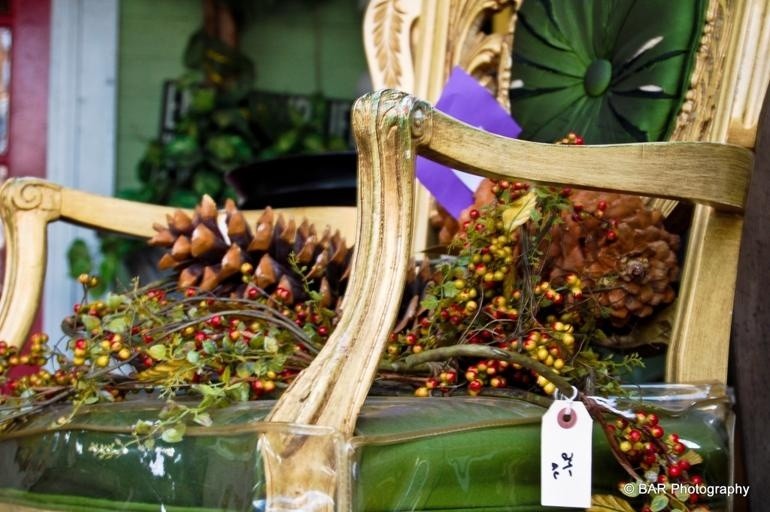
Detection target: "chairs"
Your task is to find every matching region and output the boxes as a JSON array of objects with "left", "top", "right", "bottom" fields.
[{"left": 0, "top": 0, "right": 770, "bottom": 512}]
